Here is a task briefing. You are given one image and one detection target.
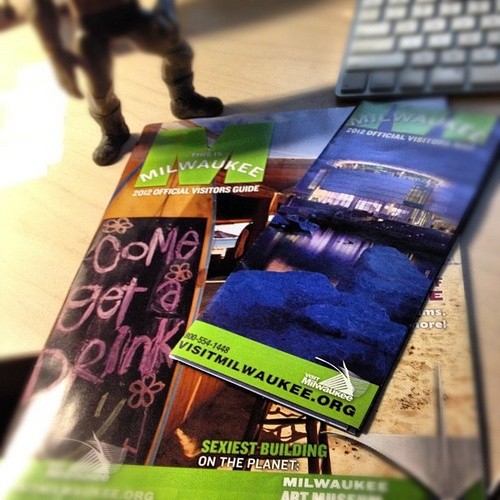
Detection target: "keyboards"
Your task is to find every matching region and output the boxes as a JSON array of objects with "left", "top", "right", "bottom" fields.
[{"left": 335, "top": 0, "right": 500, "bottom": 104}]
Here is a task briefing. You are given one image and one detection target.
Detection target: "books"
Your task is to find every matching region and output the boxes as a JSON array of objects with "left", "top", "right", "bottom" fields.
[{"left": 0, "top": 90, "right": 490, "bottom": 500}]
